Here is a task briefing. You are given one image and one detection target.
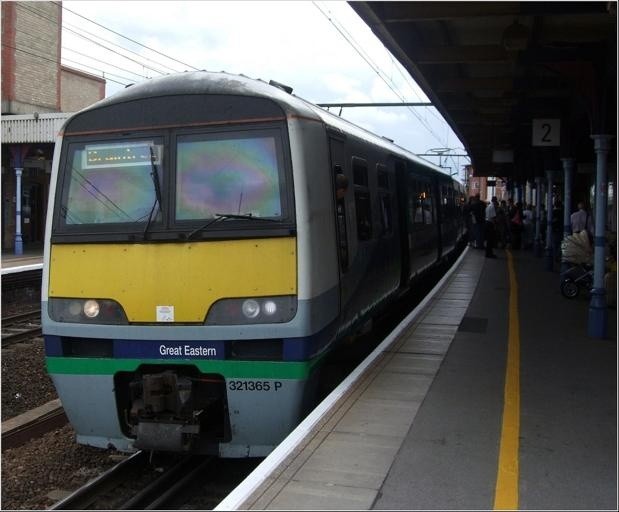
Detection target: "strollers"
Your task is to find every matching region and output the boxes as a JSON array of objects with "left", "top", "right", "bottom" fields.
[{"left": 561, "top": 231, "right": 593, "bottom": 299}]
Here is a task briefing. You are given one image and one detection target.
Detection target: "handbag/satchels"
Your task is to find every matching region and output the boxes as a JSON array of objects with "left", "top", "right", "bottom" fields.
[{"left": 512, "top": 214, "right": 522, "bottom": 225}]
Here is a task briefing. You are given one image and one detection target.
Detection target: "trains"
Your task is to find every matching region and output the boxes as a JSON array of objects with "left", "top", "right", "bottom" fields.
[{"left": 42, "top": 69, "right": 471, "bottom": 473}]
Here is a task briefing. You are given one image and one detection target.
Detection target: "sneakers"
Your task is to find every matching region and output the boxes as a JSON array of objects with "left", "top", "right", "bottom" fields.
[{"left": 485, "top": 254, "right": 498, "bottom": 259}]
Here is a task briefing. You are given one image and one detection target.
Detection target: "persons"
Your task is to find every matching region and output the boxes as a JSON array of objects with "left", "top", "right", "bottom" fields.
[
  {"left": 410, "top": 198, "right": 455, "bottom": 227},
  {"left": 459, "top": 191, "right": 617, "bottom": 259}
]
